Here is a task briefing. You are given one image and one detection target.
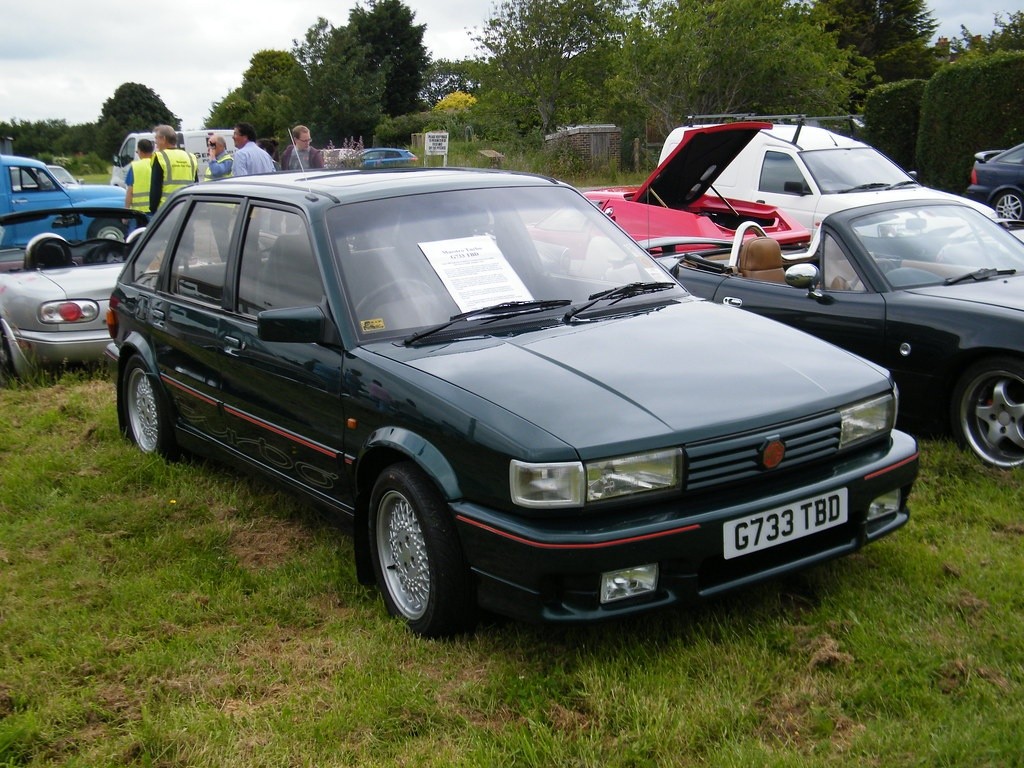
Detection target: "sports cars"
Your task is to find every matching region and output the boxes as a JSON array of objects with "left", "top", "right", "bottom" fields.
[
  {"left": 672, "top": 196, "right": 1024, "bottom": 468},
  {"left": 522, "top": 120, "right": 813, "bottom": 293},
  {"left": 1, "top": 205, "right": 215, "bottom": 376}
]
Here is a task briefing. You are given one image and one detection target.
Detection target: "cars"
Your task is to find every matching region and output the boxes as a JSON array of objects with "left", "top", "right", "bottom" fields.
[
  {"left": 103, "top": 165, "right": 923, "bottom": 638},
  {"left": 967, "top": 141, "right": 1024, "bottom": 228},
  {"left": 10, "top": 168, "right": 83, "bottom": 189},
  {"left": 1, "top": 155, "right": 130, "bottom": 251},
  {"left": 342, "top": 147, "right": 417, "bottom": 170},
  {"left": 321, "top": 149, "right": 354, "bottom": 166}
]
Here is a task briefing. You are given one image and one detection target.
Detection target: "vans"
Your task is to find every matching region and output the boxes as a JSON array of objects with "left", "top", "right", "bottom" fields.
[
  {"left": 109, "top": 130, "right": 235, "bottom": 182},
  {"left": 658, "top": 122, "right": 1000, "bottom": 240}
]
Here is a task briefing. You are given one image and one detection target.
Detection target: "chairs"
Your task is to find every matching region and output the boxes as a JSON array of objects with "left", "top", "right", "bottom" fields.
[
  {"left": 825, "top": 229, "right": 862, "bottom": 281},
  {"left": 24, "top": 233, "right": 72, "bottom": 269},
  {"left": 739, "top": 236, "right": 785, "bottom": 283},
  {"left": 123, "top": 228, "right": 146, "bottom": 260},
  {"left": 261, "top": 230, "right": 350, "bottom": 309}
]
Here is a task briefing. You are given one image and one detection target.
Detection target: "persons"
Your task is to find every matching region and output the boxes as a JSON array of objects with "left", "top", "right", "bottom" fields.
[
  {"left": 231, "top": 122, "right": 278, "bottom": 264},
  {"left": 149, "top": 125, "right": 199, "bottom": 268},
  {"left": 124, "top": 138, "right": 154, "bottom": 237},
  {"left": 281, "top": 125, "right": 324, "bottom": 235},
  {"left": 204, "top": 135, "right": 236, "bottom": 262}
]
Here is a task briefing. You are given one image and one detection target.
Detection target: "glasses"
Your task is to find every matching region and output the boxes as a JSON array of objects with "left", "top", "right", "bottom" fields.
[{"left": 297, "top": 138, "right": 313, "bottom": 144}]
